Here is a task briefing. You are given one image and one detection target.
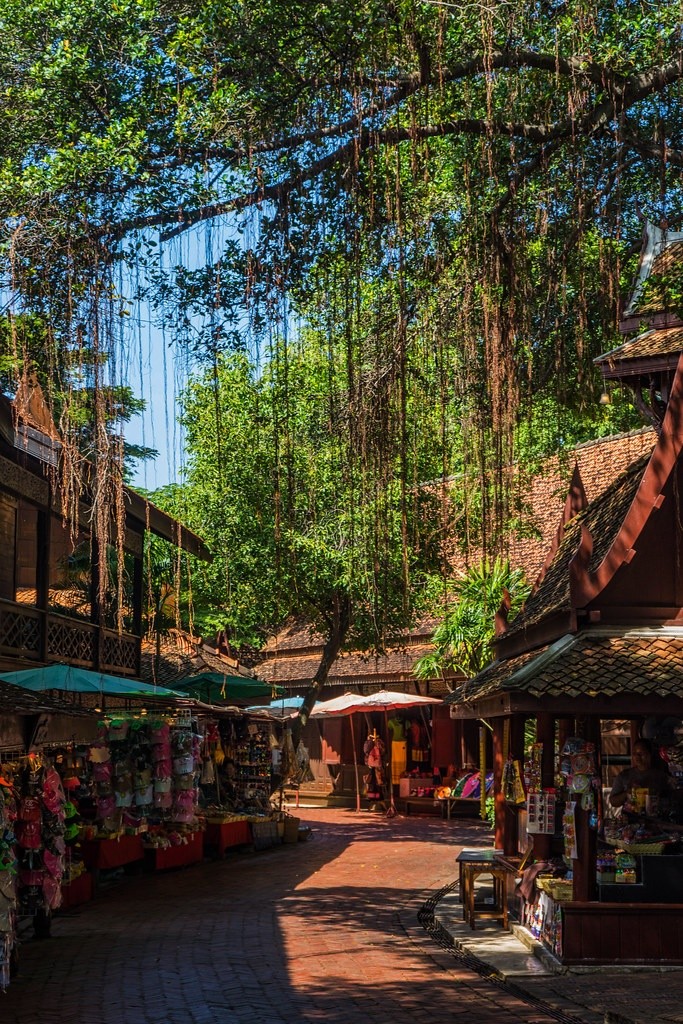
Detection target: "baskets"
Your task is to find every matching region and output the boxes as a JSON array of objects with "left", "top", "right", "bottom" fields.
[
  {"left": 616, "top": 838, "right": 630, "bottom": 849},
  {"left": 623, "top": 841, "right": 666, "bottom": 854},
  {"left": 552, "top": 887, "right": 576, "bottom": 901},
  {"left": 606, "top": 834, "right": 620, "bottom": 845},
  {"left": 536, "top": 875, "right": 564, "bottom": 892},
  {"left": 542, "top": 880, "right": 573, "bottom": 895}
]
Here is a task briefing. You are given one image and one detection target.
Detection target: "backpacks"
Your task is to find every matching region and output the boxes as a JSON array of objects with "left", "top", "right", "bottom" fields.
[{"left": 366, "top": 747, "right": 382, "bottom": 768}]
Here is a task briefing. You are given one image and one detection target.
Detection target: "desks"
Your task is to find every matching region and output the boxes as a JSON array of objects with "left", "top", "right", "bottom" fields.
[
  {"left": 405, "top": 795, "right": 512, "bottom": 931},
  {"left": 98, "top": 807, "right": 299, "bottom": 871}
]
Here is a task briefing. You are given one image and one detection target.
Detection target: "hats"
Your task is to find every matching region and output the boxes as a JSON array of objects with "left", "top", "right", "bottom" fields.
[
  {"left": 363, "top": 740, "right": 373, "bottom": 753},
  {"left": 377, "top": 739, "right": 386, "bottom": 756},
  {"left": 364, "top": 754, "right": 373, "bottom": 770},
  {"left": 1, "top": 717, "right": 247, "bottom": 933}
]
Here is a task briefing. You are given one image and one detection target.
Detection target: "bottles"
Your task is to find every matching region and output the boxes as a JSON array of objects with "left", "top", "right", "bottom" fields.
[
  {"left": 589, "top": 809, "right": 597, "bottom": 829},
  {"left": 597, "top": 852, "right": 636, "bottom": 882},
  {"left": 522, "top": 762, "right": 542, "bottom": 793},
  {"left": 625, "top": 784, "right": 659, "bottom": 818},
  {"left": 235, "top": 732, "right": 272, "bottom": 798},
  {"left": 602, "top": 814, "right": 629, "bottom": 837}
]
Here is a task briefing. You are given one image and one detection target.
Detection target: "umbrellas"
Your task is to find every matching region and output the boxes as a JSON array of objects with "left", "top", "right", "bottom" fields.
[{"left": 247, "top": 695, "right": 443, "bottom": 818}]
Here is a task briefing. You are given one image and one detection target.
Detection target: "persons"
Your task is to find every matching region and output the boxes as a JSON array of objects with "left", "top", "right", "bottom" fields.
[{"left": 609, "top": 738, "right": 676, "bottom": 818}]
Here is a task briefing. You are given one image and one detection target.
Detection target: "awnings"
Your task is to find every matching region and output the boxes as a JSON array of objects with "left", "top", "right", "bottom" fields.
[{"left": 0, "top": 665, "right": 287, "bottom": 718}]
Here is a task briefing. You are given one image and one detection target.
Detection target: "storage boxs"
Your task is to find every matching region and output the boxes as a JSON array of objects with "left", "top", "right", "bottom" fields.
[{"left": 399, "top": 774, "right": 435, "bottom": 799}]
